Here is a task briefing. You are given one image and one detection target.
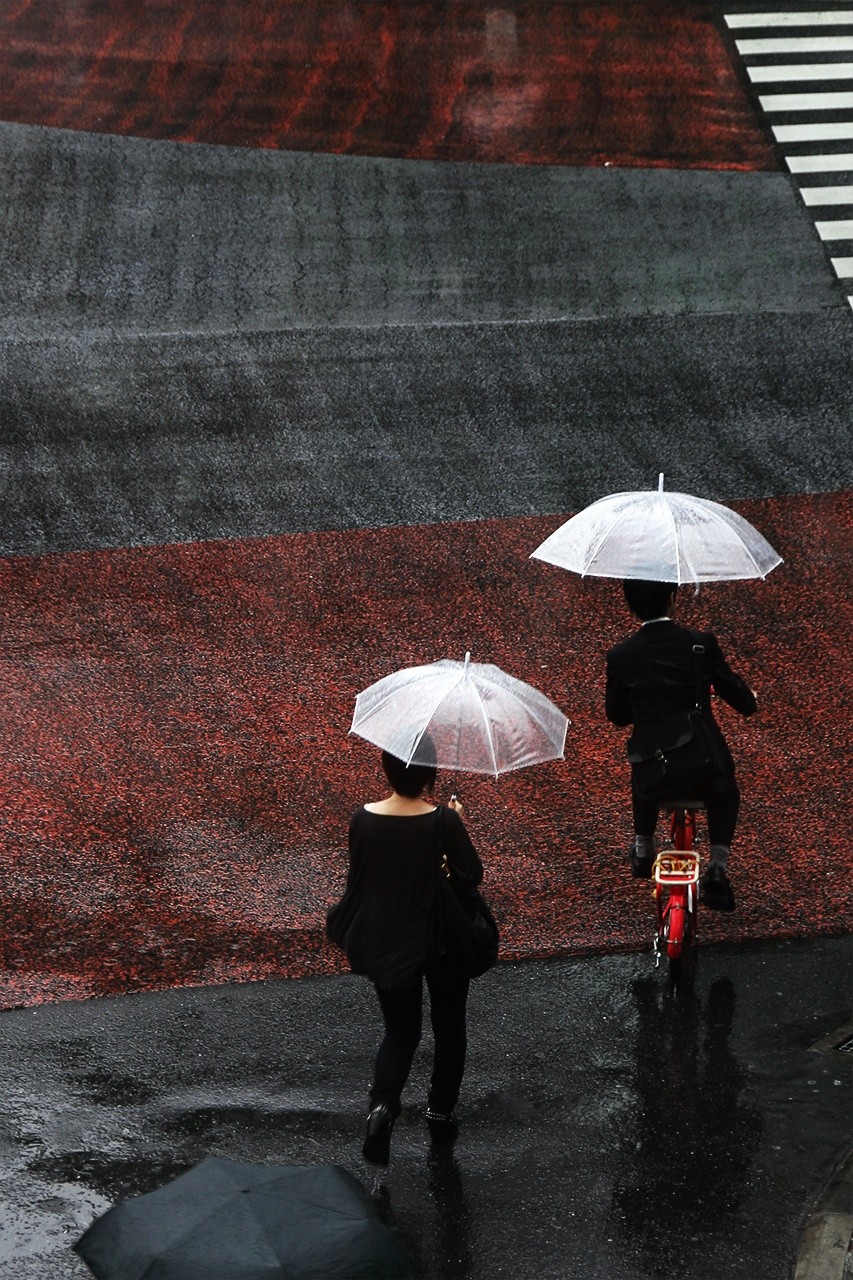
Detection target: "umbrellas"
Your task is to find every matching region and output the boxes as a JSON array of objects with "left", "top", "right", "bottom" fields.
[
  {"left": 72, "top": 1155, "right": 413, "bottom": 1279},
  {"left": 346, "top": 650, "right": 573, "bottom": 806},
  {"left": 526, "top": 471, "right": 785, "bottom": 599}
]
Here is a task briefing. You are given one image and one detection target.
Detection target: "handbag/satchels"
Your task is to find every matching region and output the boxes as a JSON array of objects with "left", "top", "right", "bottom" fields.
[
  {"left": 626, "top": 704, "right": 724, "bottom": 793},
  {"left": 383, "top": 870, "right": 500, "bottom": 983}
]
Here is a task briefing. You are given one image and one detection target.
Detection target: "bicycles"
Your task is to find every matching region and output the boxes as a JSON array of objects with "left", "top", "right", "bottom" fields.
[{"left": 645, "top": 688, "right": 758, "bottom": 1008}]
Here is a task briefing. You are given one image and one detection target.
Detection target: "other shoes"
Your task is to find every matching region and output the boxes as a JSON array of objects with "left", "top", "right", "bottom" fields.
[
  {"left": 363, "top": 1104, "right": 392, "bottom": 1167},
  {"left": 702, "top": 866, "right": 736, "bottom": 911},
  {"left": 423, "top": 1107, "right": 458, "bottom": 1136},
  {"left": 631, "top": 845, "right": 654, "bottom": 876}
]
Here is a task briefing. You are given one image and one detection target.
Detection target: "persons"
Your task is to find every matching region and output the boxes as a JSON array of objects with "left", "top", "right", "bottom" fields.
[
  {"left": 599, "top": 571, "right": 762, "bottom": 915},
  {"left": 320, "top": 724, "right": 503, "bottom": 1171}
]
